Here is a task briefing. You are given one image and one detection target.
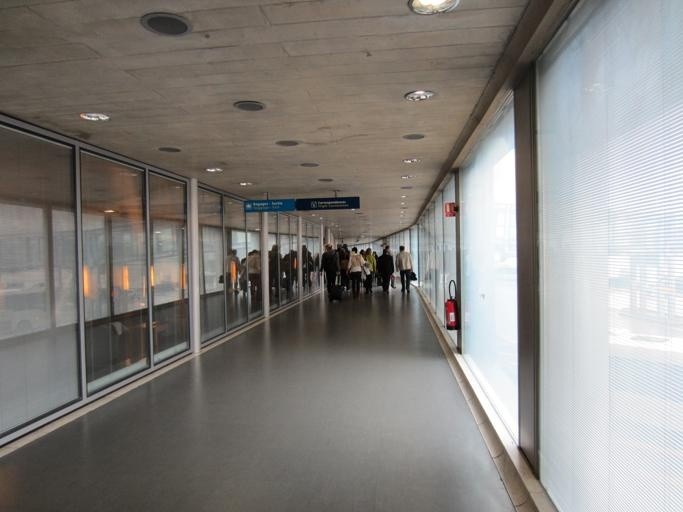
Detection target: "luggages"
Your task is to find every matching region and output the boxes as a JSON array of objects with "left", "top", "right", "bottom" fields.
[{"left": 328, "top": 275, "right": 347, "bottom": 302}]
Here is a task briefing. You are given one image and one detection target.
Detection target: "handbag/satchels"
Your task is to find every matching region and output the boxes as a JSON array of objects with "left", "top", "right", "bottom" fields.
[
  {"left": 219, "top": 274, "right": 224, "bottom": 284},
  {"left": 410, "top": 271, "right": 417, "bottom": 280}
]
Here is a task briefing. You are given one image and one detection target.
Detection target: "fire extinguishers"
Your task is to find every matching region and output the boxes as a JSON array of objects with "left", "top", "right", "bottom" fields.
[{"left": 444, "top": 280, "right": 459, "bottom": 330}]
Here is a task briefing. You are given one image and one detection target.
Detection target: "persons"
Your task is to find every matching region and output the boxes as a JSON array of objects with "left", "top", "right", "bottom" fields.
[
  {"left": 225, "top": 244, "right": 318, "bottom": 299},
  {"left": 320, "top": 243, "right": 395, "bottom": 302},
  {"left": 396, "top": 246, "right": 414, "bottom": 293}
]
